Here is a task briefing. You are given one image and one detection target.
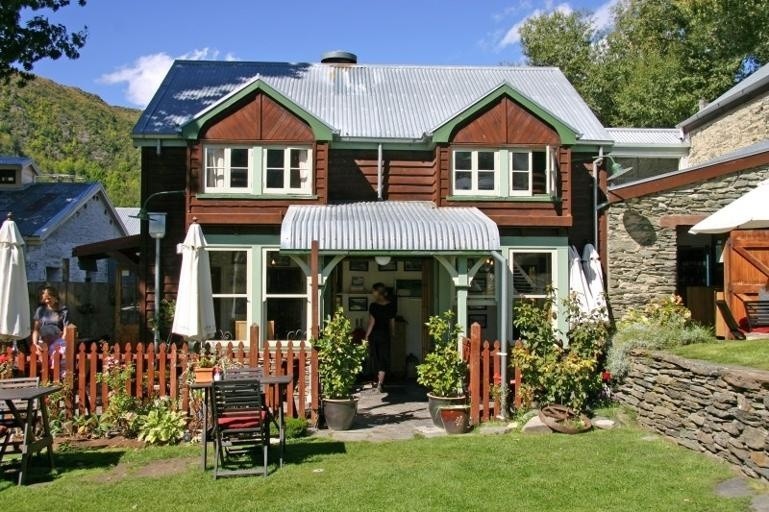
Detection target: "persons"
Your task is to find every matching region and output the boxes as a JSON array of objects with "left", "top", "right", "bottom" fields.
[
  {"left": 32, "top": 287, "right": 74, "bottom": 377},
  {"left": 363, "top": 280, "right": 398, "bottom": 395},
  {"left": 751, "top": 279, "right": 769, "bottom": 331}
]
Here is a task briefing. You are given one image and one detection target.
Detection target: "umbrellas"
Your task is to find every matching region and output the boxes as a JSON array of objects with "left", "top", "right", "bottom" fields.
[
  {"left": 0, "top": 210, "right": 33, "bottom": 343},
  {"left": 584, "top": 239, "right": 613, "bottom": 328},
  {"left": 687, "top": 176, "right": 769, "bottom": 234},
  {"left": 570, "top": 244, "right": 606, "bottom": 325},
  {"left": 168, "top": 214, "right": 218, "bottom": 349}
]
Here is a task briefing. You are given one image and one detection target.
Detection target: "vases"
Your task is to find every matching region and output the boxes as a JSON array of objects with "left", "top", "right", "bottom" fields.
[
  {"left": 193, "top": 356, "right": 213, "bottom": 384},
  {"left": 440, "top": 406, "right": 469, "bottom": 434}
]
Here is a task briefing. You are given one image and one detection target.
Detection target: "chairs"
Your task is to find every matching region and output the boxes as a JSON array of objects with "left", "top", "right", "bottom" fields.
[
  {"left": 211, "top": 368, "right": 269, "bottom": 481},
  {"left": 1, "top": 376, "right": 40, "bottom": 473}
]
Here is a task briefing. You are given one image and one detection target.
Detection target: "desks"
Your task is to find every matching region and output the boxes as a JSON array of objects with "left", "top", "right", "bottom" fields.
[
  {"left": 1, "top": 387, "right": 61, "bottom": 487},
  {"left": 189, "top": 376, "right": 291, "bottom": 471}
]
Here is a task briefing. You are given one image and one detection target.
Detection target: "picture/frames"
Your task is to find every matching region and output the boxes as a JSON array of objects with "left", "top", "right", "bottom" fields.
[
  {"left": 350, "top": 255, "right": 427, "bottom": 271},
  {"left": 455, "top": 255, "right": 488, "bottom": 329},
  {"left": 335, "top": 295, "right": 368, "bottom": 311},
  {"left": 396, "top": 279, "right": 423, "bottom": 298}
]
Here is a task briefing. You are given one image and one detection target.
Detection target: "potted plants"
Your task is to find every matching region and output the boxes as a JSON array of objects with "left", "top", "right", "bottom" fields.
[
  {"left": 309, "top": 303, "right": 369, "bottom": 430},
  {"left": 416, "top": 308, "right": 471, "bottom": 429}
]
